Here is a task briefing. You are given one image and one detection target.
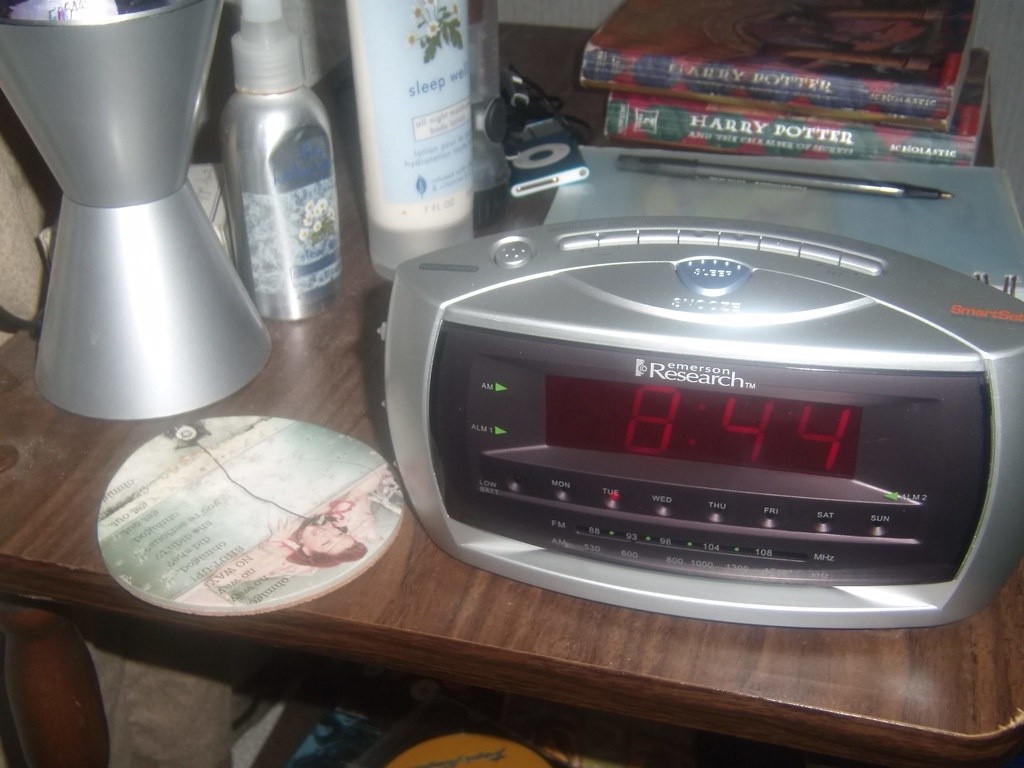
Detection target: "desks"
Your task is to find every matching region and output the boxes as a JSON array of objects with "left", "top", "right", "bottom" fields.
[{"left": 0, "top": 25, "right": 1024, "bottom": 768}]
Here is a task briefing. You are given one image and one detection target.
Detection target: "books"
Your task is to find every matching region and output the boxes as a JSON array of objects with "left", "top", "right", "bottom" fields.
[{"left": 580, "top": 0, "right": 992, "bottom": 168}]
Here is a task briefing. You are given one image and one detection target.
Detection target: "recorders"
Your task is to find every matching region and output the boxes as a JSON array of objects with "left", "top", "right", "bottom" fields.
[{"left": 375, "top": 216, "right": 1023, "bottom": 630}]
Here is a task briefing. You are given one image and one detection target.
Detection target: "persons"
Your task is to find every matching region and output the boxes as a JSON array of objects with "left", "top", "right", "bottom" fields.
[{"left": 215, "top": 470, "right": 383, "bottom": 581}]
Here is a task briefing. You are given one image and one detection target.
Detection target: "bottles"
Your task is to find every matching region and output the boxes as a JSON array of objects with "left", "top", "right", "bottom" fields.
[{"left": 217, "top": 0, "right": 344, "bottom": 322}]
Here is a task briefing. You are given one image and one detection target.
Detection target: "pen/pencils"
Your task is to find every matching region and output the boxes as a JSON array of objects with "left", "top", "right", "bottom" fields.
[{"left": 612, "top": 150, "right": 956, "bottom": 201}]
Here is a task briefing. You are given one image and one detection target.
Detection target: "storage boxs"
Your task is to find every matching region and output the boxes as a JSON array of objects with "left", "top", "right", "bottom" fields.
[{"left": 39, "top": 162, "right": 236, "bottom": 271}]
